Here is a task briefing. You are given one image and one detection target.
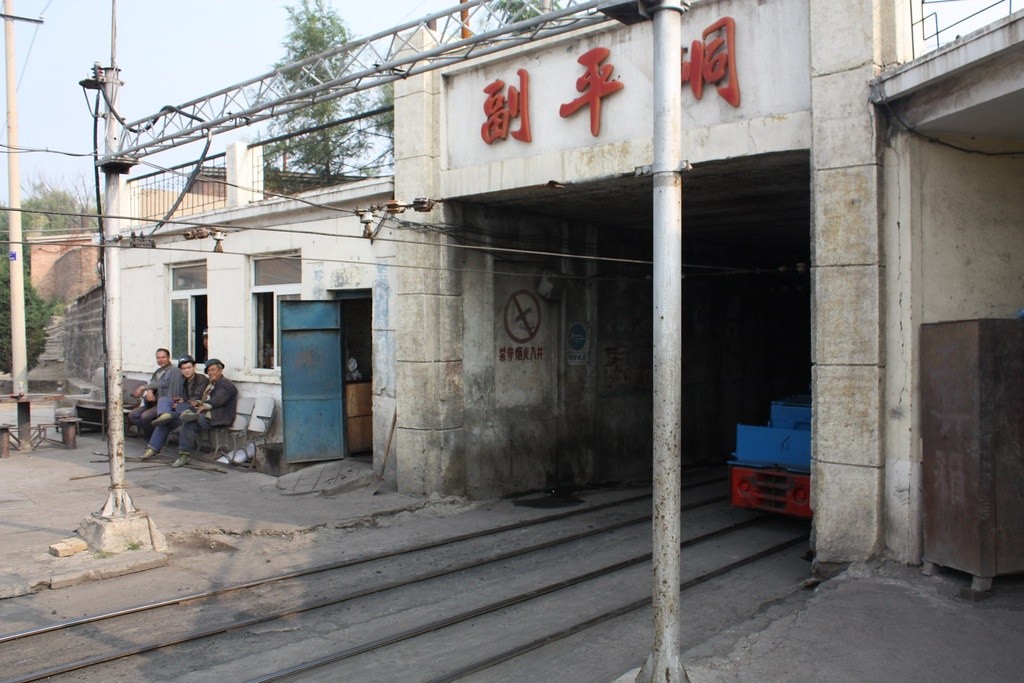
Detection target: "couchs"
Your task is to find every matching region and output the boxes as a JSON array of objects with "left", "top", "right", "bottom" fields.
[{"left": 54, "top": 367, "right": 106, "bottom": 435}]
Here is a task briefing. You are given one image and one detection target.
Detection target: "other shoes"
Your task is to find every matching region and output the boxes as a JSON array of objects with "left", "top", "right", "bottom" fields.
[
  {"left": 139, "top": 446, "right": 158, "bottom": 460},
  {"left": 179, "top": 412, "right": 198, "bottom": 423},
  {"left": 171, "top": 454, "right": 191, "bottom": 468},
  {"left": 151, "top": 413, "right": 173, "bottom": 426}
]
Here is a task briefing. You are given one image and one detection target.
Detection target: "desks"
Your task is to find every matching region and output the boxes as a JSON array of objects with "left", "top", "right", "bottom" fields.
[
  {"left": 0, "top": 394, "right": 66, "bottom": 453},
  {"left": 76, "top": 399, "right": 107, "bottom": 441}
]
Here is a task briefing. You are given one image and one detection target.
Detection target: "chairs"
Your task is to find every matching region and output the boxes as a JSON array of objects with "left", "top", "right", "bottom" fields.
[{"left": 124, "top": 378, "right": 278, "bottom": 469}]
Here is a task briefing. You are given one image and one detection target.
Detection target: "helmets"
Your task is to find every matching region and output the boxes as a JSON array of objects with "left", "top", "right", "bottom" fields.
[
  {"left": 201, "top": 328, "right": 207, "bottom": 338},
  {"left": 204, "top": 358, "right": 225, "bottom": 374},
  {"left": 177, "top": 355, "right": 196, "bottom": 369}
]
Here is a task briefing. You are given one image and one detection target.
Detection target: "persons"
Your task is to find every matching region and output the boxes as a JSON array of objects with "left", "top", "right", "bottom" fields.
[
  {"left": 171, "top": 359, "right": 238, "bottom": 467},
  {"left": 201, "top": 328, "right": 208, "bottom": 363},
  {"left": 129, "top": 348, "right": 183, "bottom": 453},
  {"left": 139, "top": 355, "right": 210, "bottom": 459}
]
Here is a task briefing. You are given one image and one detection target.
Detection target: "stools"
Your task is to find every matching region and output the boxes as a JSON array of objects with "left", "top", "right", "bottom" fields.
[{"left": 0, "top": 414, "right": 83, "bottom": 459}]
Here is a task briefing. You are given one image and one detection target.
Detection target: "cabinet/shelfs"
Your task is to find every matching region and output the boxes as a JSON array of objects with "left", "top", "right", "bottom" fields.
[
  {"left": 346, "top": 380, "right": 372, "bottom": 451},
  {"left": 921, "top": 317, "right": 1024, "bottom": 592}
]
